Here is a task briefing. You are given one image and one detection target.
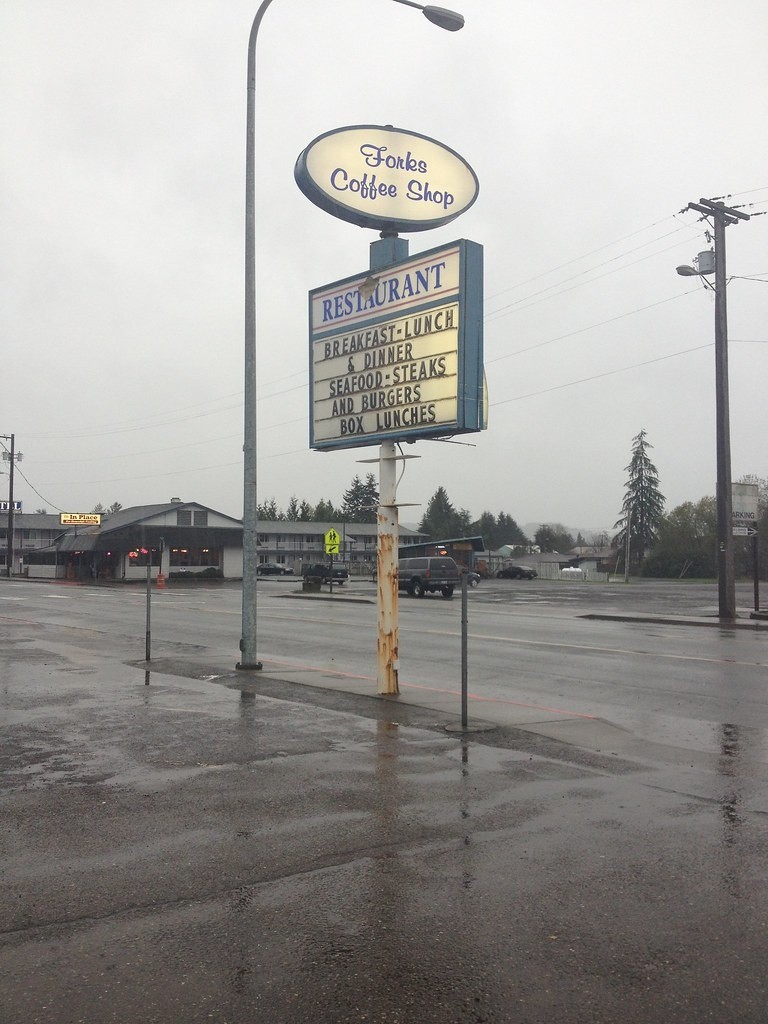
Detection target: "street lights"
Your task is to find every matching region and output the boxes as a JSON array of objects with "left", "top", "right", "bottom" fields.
[
  {"left": 235, "top": 0, "right": 467, "bottom": 673},
  {"left": 676, "top": 264, "right": 736, "bottom": 617}
]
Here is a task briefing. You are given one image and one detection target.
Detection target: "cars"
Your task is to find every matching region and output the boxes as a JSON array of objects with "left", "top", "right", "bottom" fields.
[
  {"left": 456, "top": 564, "right": 481, "bottom": 589},
  {"left": 256, "top": 563, "right": 295, "bottom": 576},
  {"left": 497, "top": 566, "right": 538, "bottom": 580}
]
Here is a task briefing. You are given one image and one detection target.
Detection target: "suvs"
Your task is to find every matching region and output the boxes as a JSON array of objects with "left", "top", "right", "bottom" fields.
[
  {"left": 302, "top": 562, "right": 349, "bottom": 586},
  {"left": 398, "top": 556, "right": 459, "bottom": 598}
]
